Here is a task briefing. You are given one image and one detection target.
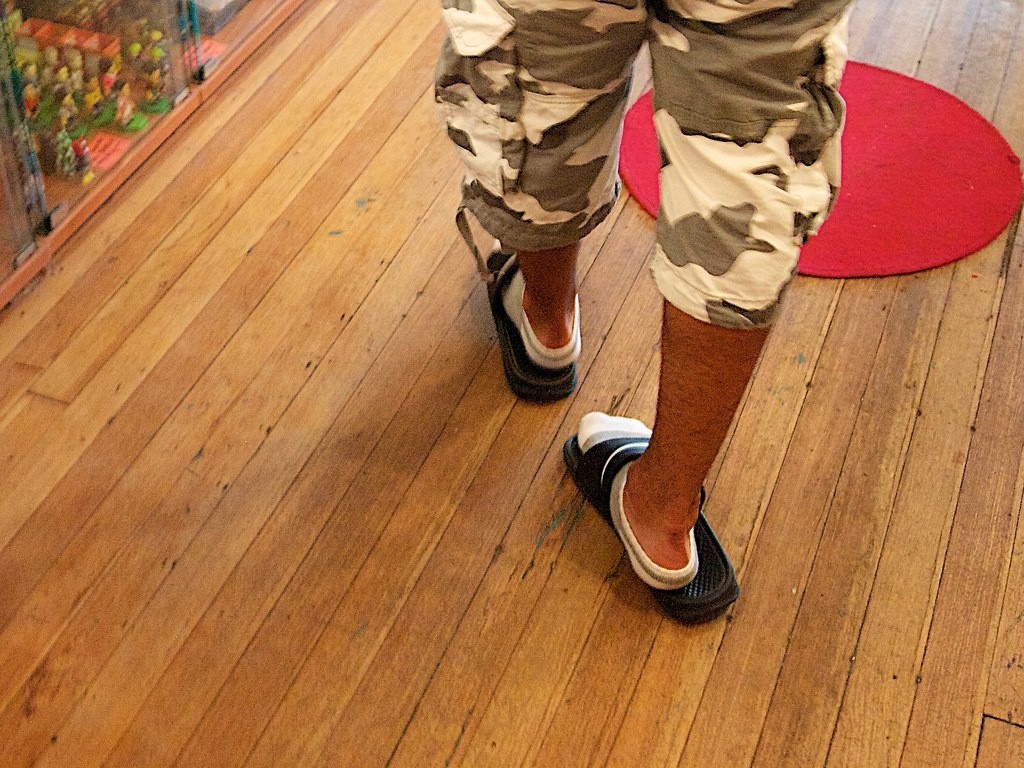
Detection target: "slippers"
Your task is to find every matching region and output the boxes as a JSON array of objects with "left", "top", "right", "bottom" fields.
[
  {"left": 562, "top": 434, "right": 741, "bottom": 624},
  {"left": 487, "top": 243, "right": 577, "bottom": 402}
]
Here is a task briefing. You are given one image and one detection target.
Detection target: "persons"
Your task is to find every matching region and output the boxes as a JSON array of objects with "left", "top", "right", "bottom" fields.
[
  {"left": 432, "top": 0, "right": 860, "bottom": 592},
  {"left": 16, "top": 29, "right": 173, "bottom": 179}
]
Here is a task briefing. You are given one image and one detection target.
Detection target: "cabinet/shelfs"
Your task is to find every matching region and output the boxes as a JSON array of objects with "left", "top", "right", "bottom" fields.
[
  {"left": 0, "top": 0, "right": 202, "bottom": 258},
  {"left": 0, "top": 88, "right": 52, "bottom": 311},
  {"left": 184, "top": 0, "right": 306, "bottom": 106}
]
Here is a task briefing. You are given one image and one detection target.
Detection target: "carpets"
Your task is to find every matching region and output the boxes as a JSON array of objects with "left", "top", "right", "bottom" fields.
[{"left": 618, "top": 60, "right": 1024, "bottom": 279}]
